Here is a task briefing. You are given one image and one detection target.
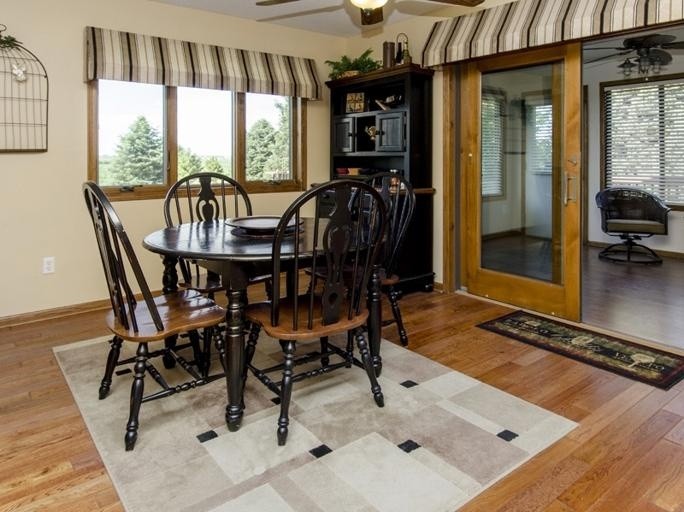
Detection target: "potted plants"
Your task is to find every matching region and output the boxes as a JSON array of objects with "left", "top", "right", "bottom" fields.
[{"left": 323, "top": 46, "right": 383, "bottom": 80}]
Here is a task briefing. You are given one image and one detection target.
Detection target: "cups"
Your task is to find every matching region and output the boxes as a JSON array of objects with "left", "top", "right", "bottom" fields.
[{"left": 383, "top": 42, "right": 396, "bottom": 68}]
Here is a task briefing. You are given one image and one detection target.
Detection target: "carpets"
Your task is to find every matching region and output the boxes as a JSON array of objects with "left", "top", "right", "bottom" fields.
[
  {"left": 474, "top": 310, "right": 684, "bottom": 391},
  {"left": 51, "top": 322, "right": 580, "bottom": 511}
]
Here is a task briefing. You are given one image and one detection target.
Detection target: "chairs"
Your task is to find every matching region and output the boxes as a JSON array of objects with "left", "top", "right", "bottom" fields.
[
  {"left": 303, "top": 171, "right": 416, "bottom": 378},
  {"left": 161, "top": 171, "right": 284, "bottom": 375},
  {"left": 595, "top": 187, "right": 672, "bottom": 266},
  {"left": 244, "top": 177, "right": 390, "bottom": 448},
  {"left": 83, "top": 180, "right": 247, "bottom": 453}
]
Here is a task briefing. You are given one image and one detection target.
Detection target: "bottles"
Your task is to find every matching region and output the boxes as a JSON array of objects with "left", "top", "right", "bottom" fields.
[{"left": 396, "top": 42, "right": 412, "bottom": 66}]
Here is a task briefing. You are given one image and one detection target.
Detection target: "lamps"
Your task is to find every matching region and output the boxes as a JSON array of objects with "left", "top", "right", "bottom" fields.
[
  {"left": 351, "top": 0, "right": 390, "bottom": 18},
  {"left": 618, "top": 56, "right": 663, "bottom": 76}
]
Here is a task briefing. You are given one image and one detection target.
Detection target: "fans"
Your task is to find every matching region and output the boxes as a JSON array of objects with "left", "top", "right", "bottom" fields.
[
  {"left": 584, "top": 33, "right": 684, "bottom": 65},
  {"left": 256, "top": 0, "right": 486, "bottom": 26}
]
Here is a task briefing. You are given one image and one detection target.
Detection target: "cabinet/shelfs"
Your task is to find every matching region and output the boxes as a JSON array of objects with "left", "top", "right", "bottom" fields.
[{"left": 318, "top": 62, "right": 436, "bottom": 298}]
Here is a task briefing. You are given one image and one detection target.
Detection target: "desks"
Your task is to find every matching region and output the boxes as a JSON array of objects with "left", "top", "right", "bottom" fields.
[{"left": 142, "top": 216, "right": 388, "bottom": 433}]
